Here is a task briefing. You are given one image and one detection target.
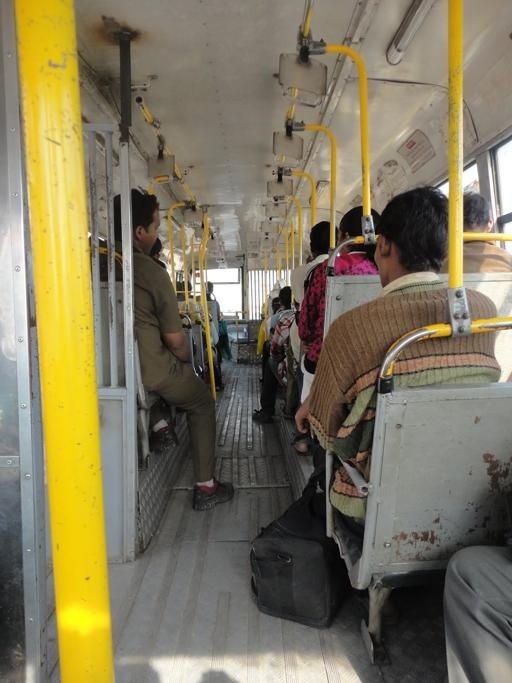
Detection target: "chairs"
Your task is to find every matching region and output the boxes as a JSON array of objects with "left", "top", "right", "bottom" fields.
[
  {"left": 91, "top": 244, "right": 223, "bottom": 470},
  {"left": 319, "top": 232, "right": 443, "bottom": 429},
  {"left": 320, "top": 314, "right": 510, "bottom": 664}
]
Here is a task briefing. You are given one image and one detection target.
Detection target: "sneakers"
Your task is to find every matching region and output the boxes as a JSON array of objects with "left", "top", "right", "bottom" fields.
[
  {"left": 193, "top": 482, "right": 234, "bottom": 511},
  {"left": 149, "top": 426, "right": 177, "bottom": 450},
  {"left": 252, "top": 409, "right": 266, "bottom": 423}
]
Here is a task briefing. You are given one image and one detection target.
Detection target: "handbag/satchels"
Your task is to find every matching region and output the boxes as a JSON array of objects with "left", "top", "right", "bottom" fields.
[{"left": 251, "top": 495, "right": 348, "bottom": 628}]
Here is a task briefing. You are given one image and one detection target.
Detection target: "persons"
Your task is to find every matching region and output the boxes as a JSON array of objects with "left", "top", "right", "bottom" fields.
[
  {"left": 294, "top": 186, "right": 512, "bottom": 537},
  {"left": 295, "top": 205, "right": 381, "bottom": 455},
  {"left": 253, "top": 221, "right": 340, "bottom": 423},
  {"left": 443, "top": 545, "right": 512, "bottom": 683},
  {"left": 99, "top": 189, "right": 234, "bottom": 511},
  {"left": 178, "top": 280, "right": 224, "bottom": 392},
  {"left": 441, "top": 191, "right": 512, "bottom": 274}
]
